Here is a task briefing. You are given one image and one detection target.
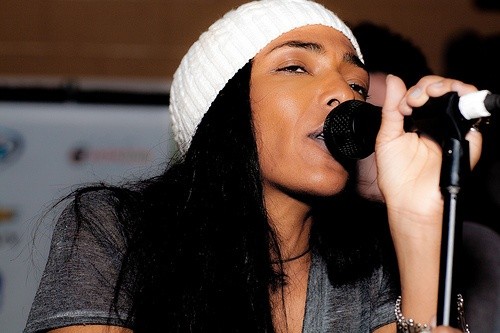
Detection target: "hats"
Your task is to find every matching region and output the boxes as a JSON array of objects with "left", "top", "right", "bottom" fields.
[{"left": 169, "top": 0, "right": 364, "bottom": 161}]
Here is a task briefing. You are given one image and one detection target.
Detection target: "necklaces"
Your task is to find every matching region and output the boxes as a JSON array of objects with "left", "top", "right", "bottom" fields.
[{"left": 271, "top": 247, "right": 308, "bottom": 263}]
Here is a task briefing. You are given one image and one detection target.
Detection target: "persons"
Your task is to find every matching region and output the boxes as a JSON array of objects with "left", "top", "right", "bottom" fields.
[
  {"left": 22, "top": 1, "right": 484, "bottom": 333},
  {"left": 342, "top": 19, "right": 500, "bottom": 333}
]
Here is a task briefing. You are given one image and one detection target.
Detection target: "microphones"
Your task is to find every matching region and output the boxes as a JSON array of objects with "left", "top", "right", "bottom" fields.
[{"left": 323, "top": 90, "right": 500, "bottom": 162}]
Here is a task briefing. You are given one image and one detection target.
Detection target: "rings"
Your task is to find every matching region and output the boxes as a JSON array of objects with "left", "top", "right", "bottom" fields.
[{"left": 470, "top": 127, "right": 480, "bottom": 131}]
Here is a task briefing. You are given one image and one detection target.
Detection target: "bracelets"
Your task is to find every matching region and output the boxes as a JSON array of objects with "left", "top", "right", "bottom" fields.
[{"left": 392, "top": 294, "right": 471, "bottom": 333}]
